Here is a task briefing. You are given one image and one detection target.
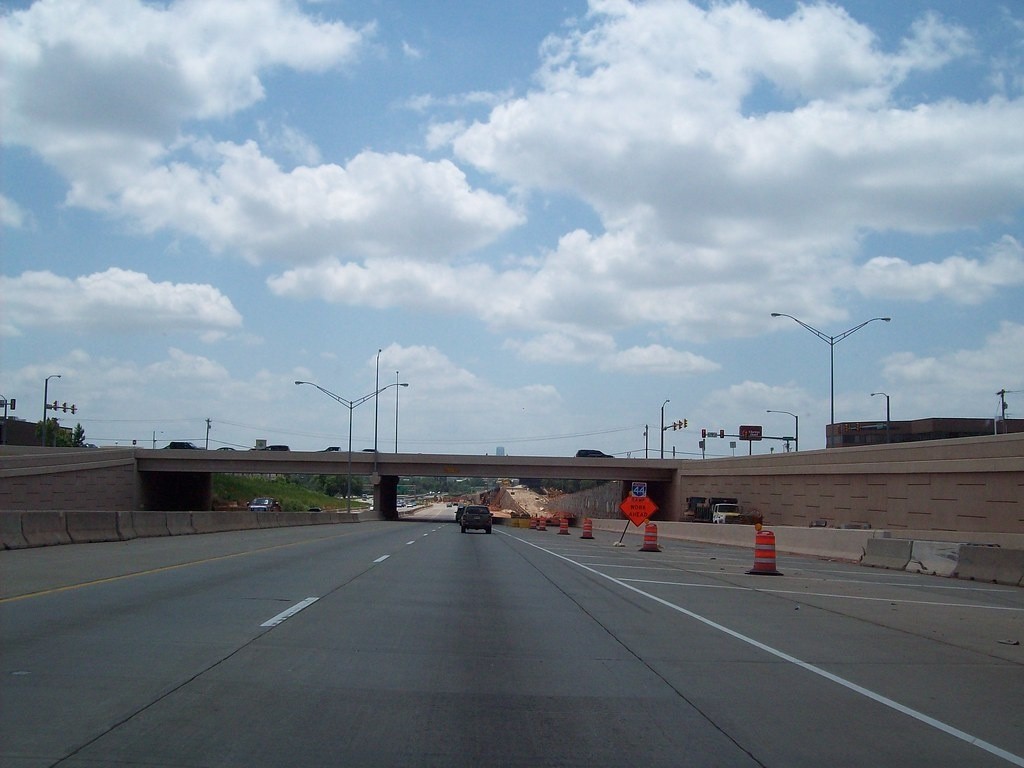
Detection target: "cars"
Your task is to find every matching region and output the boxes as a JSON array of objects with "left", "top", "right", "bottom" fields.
[
  {"left": 397, "top": 500, "right": 406, "bottom": 507},
  {"left": 446, "top": 503, "right": 452, "bottom": 507},
  {"left": 453, "top": 502, "right": 458, "bottom": 506},
  {"left": 407, "top": 501, "right": 413, "bottom": 507},
  {"left": 307, "top": 509, "right": 321, "bottom": 513},
  {"left": 81, "top": 443, "right": 100, "bottom": 448}
]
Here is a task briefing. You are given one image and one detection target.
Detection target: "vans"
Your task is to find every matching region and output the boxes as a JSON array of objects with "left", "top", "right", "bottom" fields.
[{"left": 171, "top": 441, "right": 200, "bottom": 449}]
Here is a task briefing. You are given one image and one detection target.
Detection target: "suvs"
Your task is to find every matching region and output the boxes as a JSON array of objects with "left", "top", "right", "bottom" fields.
[
  {"left": 460, "top": 505, "right": 493, "bottom": 534},
  {"left": 265, "top": 445, "right": 289, "bottom": 451},
  {"left": 248, "top": 498, "right": 282, "bottom": 512},
  {"left": 576, "top": 448, "right": 614, "bottom": 457},
  {"left": 455, "top": 507, "right": 465, "bottom": 523}
]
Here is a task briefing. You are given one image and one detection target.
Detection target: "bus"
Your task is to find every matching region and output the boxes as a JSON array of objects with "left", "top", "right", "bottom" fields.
[{"left": 410, "top": 498, "right": 416, "bottom": 506}]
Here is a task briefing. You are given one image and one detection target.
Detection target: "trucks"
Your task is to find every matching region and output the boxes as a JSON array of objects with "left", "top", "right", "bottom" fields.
[
  {"left": 683, "top": 497, "right": 707, "bottom": 521},
  {"left": 694, "top": 497, "right": 745, "bottom": 524}
]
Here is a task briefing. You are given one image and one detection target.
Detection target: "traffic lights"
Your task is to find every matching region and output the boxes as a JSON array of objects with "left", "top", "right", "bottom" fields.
[
  {"left": 720, "top": 430, "right": 724, "bottom": 438},
  {"left": 702, "top": 429, "right": 706, "bottom": 438},
  {"left": 674, "top": 422, "right": 677, "bottom": 431},
  {"left": 53, "top": 401, "right": 57, "bottom": 411},
  {"left": 684, "top": 419, "right": 687, "bottom": 428},
  {"left": 62, "top": 403, "right": 66, "bottom": 413},
  {"left": 679, "top": 420, "right": 683, "bottom": 429},
  {"left": 71, "top": 405, "right": 74, "bottom": 414}
]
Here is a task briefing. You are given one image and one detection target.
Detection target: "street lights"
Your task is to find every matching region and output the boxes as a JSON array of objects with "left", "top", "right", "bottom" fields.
[
  {"left": 42, "top": 375, "right": 61, "bottom": 445},
  {"left": 767, "top": 410, "right": 798, "bottom": 452},
  {"left": 375, "top": 349, "right": 382, "bottom": 507},
  {"left": 661, "top": 400, "right": 669, "bottom": 460},
  {"left": 871, "top": 392, "right": 889, "bottom": 444},
  {"left": 770, "top": 313, "right": 892, "bottom": 446},
  {"left": 295, "top": 381, "right": 409, "bottom": 512},
  {"left": 396, "top": 371, "right": 399, "bottom": 454}
]
